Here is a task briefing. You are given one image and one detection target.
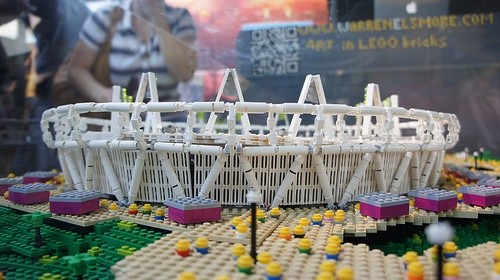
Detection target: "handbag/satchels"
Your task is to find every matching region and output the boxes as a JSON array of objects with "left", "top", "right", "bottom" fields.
[{"left": 50, "top": 38, "right": 113, "bottom": 131}]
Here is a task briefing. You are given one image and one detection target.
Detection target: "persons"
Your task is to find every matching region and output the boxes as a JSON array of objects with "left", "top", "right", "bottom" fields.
[
  {"left": 24, "top": 75, "right": 58, "bottom": 171},
  {"left": 65, "top": 0, "right": 200, "bottom": 121}
]
